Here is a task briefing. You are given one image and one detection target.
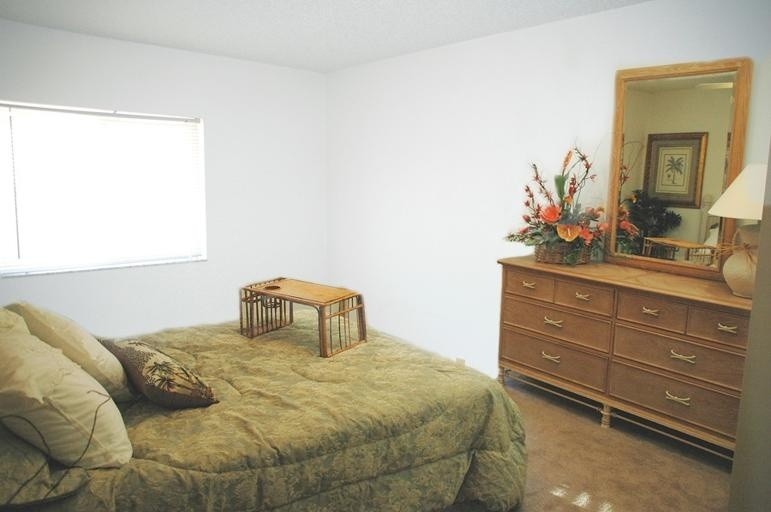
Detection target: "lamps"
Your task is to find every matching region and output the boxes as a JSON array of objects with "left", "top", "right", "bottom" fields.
[{"left": 708, "top": 162, "right": 768, "bottom": 298}]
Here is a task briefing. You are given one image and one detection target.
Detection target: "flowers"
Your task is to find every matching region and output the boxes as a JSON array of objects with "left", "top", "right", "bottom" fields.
[
  {"left": 504, "top": 142, "right": 608, "bottom": 265},
  {"left": 616, "top": 142, "right": 645, "bottom": 253}
]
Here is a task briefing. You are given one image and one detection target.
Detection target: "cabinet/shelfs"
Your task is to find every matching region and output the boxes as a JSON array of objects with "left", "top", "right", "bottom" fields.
[{"left": 496, "top": 264, "right": 749, "bottom": 461}]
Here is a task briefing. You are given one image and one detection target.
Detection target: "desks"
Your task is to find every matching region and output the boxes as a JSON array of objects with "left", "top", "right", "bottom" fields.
[
  {"left": 238, "top": 275, "right": 366, "bottom": 357},
  {"left": 641, "top": 236, "right": 715, "bottom": 263}
]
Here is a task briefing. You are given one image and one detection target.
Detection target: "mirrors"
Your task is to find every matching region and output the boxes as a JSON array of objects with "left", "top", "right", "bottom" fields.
[{"left": 601, "top": 56, "right": 751, "bottom": 282}]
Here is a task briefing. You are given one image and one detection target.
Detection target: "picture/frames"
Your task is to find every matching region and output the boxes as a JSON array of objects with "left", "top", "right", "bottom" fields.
[{"left": 643, "top": 132, "right": 709, "bottom": 209}]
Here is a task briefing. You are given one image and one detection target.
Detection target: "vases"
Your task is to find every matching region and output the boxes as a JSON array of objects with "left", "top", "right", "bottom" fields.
[{"left": 534, "top": 237, "right": 594, "bottom": 265}]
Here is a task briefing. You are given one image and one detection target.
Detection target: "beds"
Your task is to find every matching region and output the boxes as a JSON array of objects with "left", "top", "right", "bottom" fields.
[{"left": 0, "top": 311, "right": 529, "bottom": 512}]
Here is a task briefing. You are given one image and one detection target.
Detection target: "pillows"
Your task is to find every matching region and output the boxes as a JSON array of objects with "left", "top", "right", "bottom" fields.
[
  {"left": 0, "top": 307, "right": 133, "bottom": 469},
  {"left": 4, "top": 302, "right": 140, "bottom": 402},
  {"left": 96, "top": 335, "right": 220, "bottom": 409}
]
